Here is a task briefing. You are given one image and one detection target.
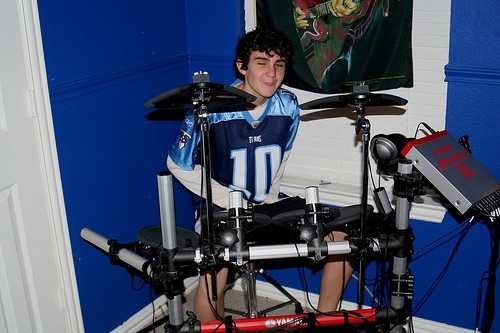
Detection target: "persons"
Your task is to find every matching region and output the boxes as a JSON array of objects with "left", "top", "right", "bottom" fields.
[{"left": 170, "top": 25, "right": 357, "bottom": 322}]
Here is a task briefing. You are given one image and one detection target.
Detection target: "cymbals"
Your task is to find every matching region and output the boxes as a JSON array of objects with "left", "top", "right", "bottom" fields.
[
  {"left": 145, "top": 81, "right": 257, "bottom": 108},
  {"left": 299, "top": 93, "right": 409, "bottom": 107}
]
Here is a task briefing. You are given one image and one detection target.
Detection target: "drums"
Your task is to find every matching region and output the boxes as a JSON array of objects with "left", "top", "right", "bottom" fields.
[
  {"left": 329, "top": 203, "right": 375, "bottom": 238},
  {"left": 136, "top": 224, "right": 202, "bottom": 279},
  {"left": 198, "top": 211, "right": 272, "bottom": 246},
  {"left": 272, "top": 207, "right": 340, "bottom": 244}
]
